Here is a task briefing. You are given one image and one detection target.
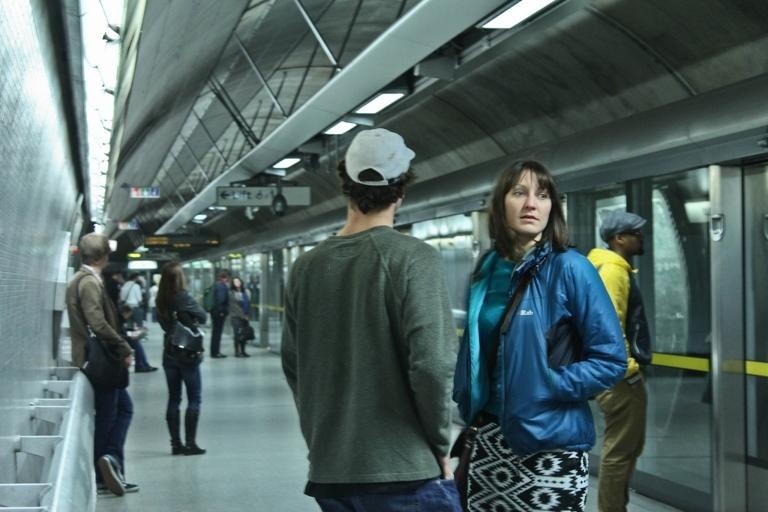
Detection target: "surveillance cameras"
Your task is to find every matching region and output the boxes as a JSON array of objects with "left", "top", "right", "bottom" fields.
[{"left": 272, "top": 195, "right": 286, "bottom": 216}]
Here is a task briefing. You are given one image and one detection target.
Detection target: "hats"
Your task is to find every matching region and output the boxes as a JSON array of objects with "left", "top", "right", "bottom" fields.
[
  {"left": 344, "top": 127, "right": 416, "bottom": 187},
  {"left": 599, "top": 209, "right": 647, "bottom": 243}
]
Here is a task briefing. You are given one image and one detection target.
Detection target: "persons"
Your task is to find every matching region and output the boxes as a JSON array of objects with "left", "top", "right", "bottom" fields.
[
  {"left": 229, "top": 277, "right": 251, "bottom": 357},
  {"left": 280, "top": 127, "right": 465, "bottom": 512},
  {"left": 156, "top": 261, "right": 205, "bottom": 455},
  {"left": 451, "top": 160, "right": 627, "bottom": 512},
  {"left": 65, "top": 232, "right": 139, "bottom": 497},
  {"left": 247, "top": 274, "right": 260, "bottom": 321},
  {"left": 584, "top": 211, "right": 651, "bottom": 512},
  {"left": 110, "top": 272, "right": 162, "bottom": 372},
  {"left": 210, "top": 269, "right": 231, "bottom": 357}
]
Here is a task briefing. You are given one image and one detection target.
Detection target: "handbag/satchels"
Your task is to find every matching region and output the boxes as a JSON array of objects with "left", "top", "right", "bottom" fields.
[
  {"left": 236, "top": 321, "right": 255, "bottom": 342},
  {"left": 450, "top": 418, "right": 478, "bottom": 508},
  {"left": 165, "top": 320, "right": 204, "bottom": 366},
  {"left": 80, "top": 334, "right": 129, "bottom": 390}
]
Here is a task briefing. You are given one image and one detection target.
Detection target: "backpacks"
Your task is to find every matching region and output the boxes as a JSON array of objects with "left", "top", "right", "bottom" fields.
[{"left": 202, "top": 282, "right": 223, "bottom": 312}]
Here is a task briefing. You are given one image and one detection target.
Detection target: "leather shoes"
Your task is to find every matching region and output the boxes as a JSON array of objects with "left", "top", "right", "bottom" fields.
[
  {"left": 184, "top": 440, "right": 206, "bottom": 456},
  {"left": 134, "top": 364, "right": 158, "bottom": 373},
  {"left": 172, "top": 439, "right": 186, "bottom": 454},
  {"left": 211, "top": 349, "right": 251, "bottom": 359}
]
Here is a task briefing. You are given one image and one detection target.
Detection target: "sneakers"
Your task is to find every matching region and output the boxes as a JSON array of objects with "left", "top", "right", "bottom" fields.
[
  {"left": 97, "top": 480, "right": 139, "bottom": 494},
  {"left": 96, "top": 453, "right": 126, "bottom": 497}
]
